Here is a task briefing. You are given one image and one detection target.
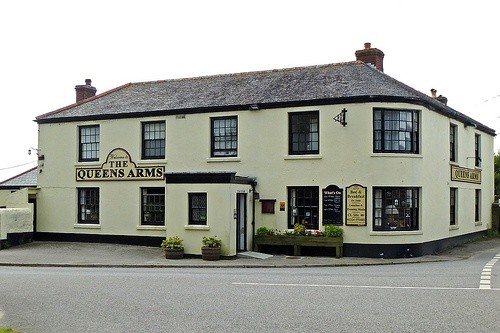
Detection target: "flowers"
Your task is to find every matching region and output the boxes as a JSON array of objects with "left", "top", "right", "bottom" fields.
[{"left": 294, "top": 223, "right": 321, "bottom": 237}]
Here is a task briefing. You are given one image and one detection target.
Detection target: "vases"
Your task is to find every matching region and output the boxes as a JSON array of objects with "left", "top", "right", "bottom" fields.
[{"left": 255, "top": 235, "right": 343, "bottom": 257}]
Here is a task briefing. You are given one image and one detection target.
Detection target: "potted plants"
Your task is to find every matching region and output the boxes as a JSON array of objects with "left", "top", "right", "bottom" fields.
[
  {"left": 201, "top": 235, "right": 222, "bottom": 260},
  {"left": 161, "top": 236, "right": 184, "bottom": 259}
]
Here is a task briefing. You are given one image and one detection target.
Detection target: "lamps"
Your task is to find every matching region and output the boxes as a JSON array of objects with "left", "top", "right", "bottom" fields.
[
  {"left": 249, "top": 188, "right": 259, "bottom": 199},
  {"left": 28, "top": 150, "right": 31, "bottom": 155}
]
